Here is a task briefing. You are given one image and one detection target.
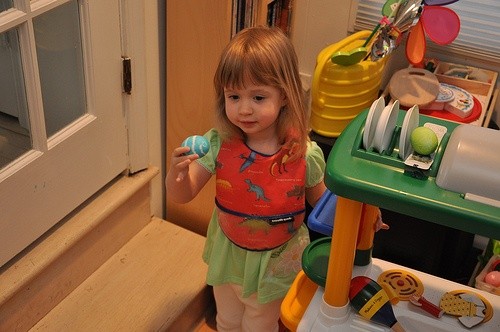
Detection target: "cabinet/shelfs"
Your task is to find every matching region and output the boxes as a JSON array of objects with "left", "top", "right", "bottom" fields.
[{"left": 165, "top": 1, "right": 293, "bottom": 235}]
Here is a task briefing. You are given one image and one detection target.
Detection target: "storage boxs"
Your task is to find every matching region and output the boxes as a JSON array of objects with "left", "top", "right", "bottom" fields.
[{"left": 278, "top": 258, "right": 499, "bottom": 331}]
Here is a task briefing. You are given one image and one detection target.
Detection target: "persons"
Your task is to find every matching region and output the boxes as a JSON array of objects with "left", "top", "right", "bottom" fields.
[{"left": 168, "top": 26, "right": 390, "bottom": 332}]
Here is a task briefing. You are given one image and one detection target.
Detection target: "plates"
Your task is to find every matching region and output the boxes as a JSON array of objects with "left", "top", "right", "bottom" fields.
[
  {"left": 362, "top": 96, "right": 385, "bottom": 150},
  {"left": 374, "top": 100, "right": 400, "bottom": 155},
  {"left": 398, "top": 104, "right": 420, "bottom": 160}
]
